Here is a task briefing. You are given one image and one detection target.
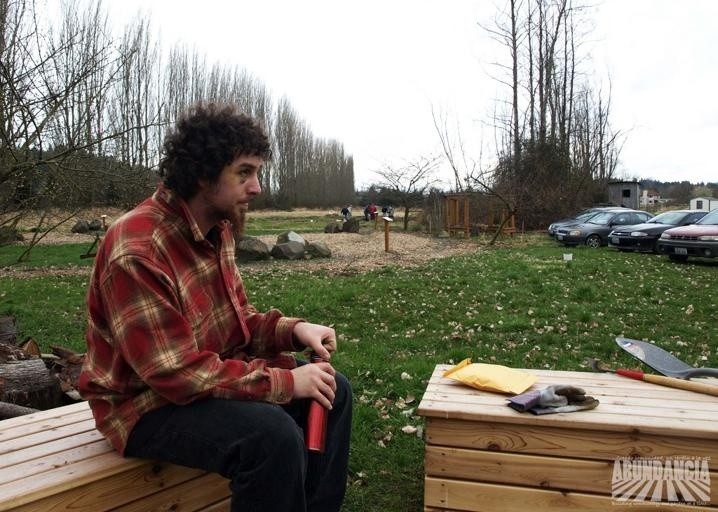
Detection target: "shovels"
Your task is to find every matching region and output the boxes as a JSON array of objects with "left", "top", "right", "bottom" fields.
[{"left": 615, "top": 337, "right": 718, "bottom": 380}]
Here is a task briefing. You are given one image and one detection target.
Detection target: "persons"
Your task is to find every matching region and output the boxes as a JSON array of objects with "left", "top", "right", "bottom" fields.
[
  {"left": 382, "top": 206, "right": 387, "bottom": 217},
  {"left": 75, "top": 103, "right": 353, "bottom": 511},
  {"left": 387, "top": 205, "right": 395, "bottom": 218},
  {"left": 365, "top": 206, "right": 370, "bottom": 220},
  {"left": 345, "top": 205, "right": 353, "bottom": 222},
  {"left": 368, "top": 203, "right": 377, "bottom": 220}
]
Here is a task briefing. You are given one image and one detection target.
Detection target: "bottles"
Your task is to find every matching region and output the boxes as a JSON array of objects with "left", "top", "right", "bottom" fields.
[{"left": 305, "top": 348, "right": 332, "bottom": 453}]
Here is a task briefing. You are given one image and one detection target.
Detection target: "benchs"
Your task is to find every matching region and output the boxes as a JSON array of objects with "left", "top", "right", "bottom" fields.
[
  {"left": 444, "top": 224, "right": 515, "bottom": 239},
  {"left": 0, "top": 399, "right": 233, "bottom": 512},
  {"left": 416, "top": 363, "right": 718, "bottom": 512}
]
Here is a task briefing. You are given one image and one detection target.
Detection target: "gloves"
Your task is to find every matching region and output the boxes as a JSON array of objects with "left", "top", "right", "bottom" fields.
[{"left": 504, "top": 384, "right": 601, "bottom": 415}]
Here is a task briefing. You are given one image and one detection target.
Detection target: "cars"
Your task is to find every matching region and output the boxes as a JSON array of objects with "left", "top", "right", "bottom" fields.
[{"left": 548, "top": 207, "right": 718, "bottom": 262}]
